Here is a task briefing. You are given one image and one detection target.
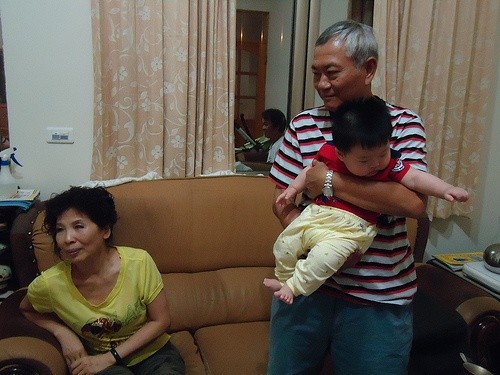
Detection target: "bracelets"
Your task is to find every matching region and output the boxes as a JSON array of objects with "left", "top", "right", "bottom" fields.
[{"left": 109, "top": 348, "right": 121, "bottom": 363}]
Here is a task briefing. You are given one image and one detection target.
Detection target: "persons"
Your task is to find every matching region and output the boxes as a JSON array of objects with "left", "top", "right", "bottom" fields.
[
  {"left": 20, "top": 183, "right": 185, "bottom": 375},
  {"left": 263, "top": 96, "right": 468, "bottom": 304},
  {"left": 245, "top": 109, "right": 287, "bottom": 171},
  {"left": 268, "top": 20, "right": 428, "bottom": 375}
]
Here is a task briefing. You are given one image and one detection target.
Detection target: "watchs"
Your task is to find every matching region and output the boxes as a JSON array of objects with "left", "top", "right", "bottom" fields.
[{"left": 321, "top": 169, "right": 334, "bottom": 198}]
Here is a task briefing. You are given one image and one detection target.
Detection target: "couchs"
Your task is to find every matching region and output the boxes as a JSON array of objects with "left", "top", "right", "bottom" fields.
[{"left": 0, "top": 175, "right": 500, "bottom": 375}]
